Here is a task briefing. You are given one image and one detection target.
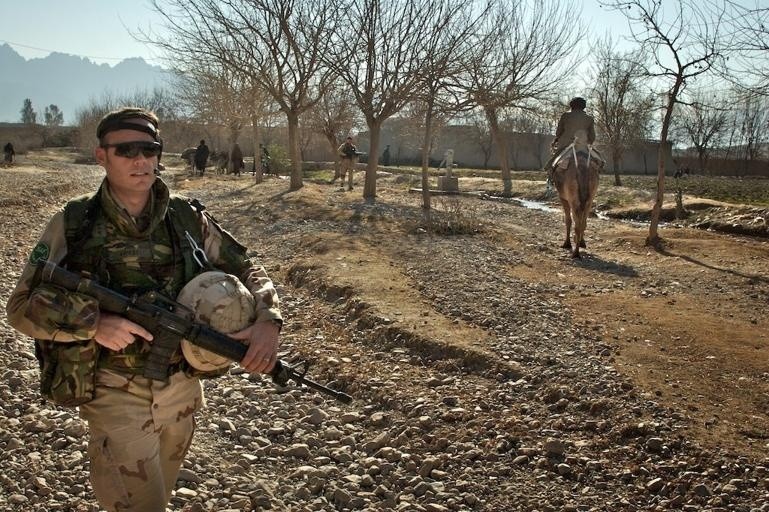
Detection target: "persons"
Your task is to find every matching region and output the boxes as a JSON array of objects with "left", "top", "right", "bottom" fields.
[
  {"left": 336, "top": 136, "right": 358, "bottom": 192},
  {"left": 230, "top": 142, "right": 244, "bottom": 177},
  {"left": 194, "top": 138, "right": 210, "bottom": 176},
  {"left": 219, "top": 149, "right": 229, "bottom": 174},
  {"left": 6, "top": 106, "right": 285, "bottom": 511},
  {"left": 542, "top": 96, "right": 607, "bottom": 175},
  {"left": 251, "top": 143, "right": 269, "bottom": 176},
  {"left": 5, "top": 142, "right": 16, "bottom": 163},
  {"left": 382, "top": 144, "right": 392, "bottom": 165}
]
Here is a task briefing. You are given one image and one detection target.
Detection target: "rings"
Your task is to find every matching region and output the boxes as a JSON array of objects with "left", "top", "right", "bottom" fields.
[{"left": 262, "top": 358, "right": 270, "bottom": 362}]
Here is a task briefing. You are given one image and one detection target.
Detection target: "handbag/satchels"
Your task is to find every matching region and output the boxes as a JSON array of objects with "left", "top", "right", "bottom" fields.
[{"left": 23, "top": 282, "right": 100, "bottom": 409}]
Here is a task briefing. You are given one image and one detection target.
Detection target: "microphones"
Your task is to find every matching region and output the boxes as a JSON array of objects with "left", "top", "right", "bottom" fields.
[{"left": 154, "top": 169, "right": 161, "bottom": 176}]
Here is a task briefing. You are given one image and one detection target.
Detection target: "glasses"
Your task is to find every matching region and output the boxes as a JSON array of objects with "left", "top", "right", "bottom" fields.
[{"left": 101, "top": 140, "right": 162, "bottom": 159}]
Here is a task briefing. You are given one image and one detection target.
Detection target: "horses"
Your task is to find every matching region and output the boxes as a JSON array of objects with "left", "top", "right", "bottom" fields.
[{"left": 552, "top": 150, "right": 600, "bottom": 258}]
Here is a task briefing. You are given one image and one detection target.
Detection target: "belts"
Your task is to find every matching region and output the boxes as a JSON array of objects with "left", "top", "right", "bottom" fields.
[{"left": 108, "top": 360, "right": 186, "bottom": 380}]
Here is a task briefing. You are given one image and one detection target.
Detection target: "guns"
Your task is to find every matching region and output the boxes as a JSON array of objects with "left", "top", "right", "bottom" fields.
[
  {"left": 340, "top": 146, "right": 367, "bottom": 160},
  {"left": 26, "top": 258, "right": 353, "bottom": 405}
]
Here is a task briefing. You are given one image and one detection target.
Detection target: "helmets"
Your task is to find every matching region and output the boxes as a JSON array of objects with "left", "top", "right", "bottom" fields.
[{"left": 173, "top": 270, "right": 259, "bottom": 374}]
